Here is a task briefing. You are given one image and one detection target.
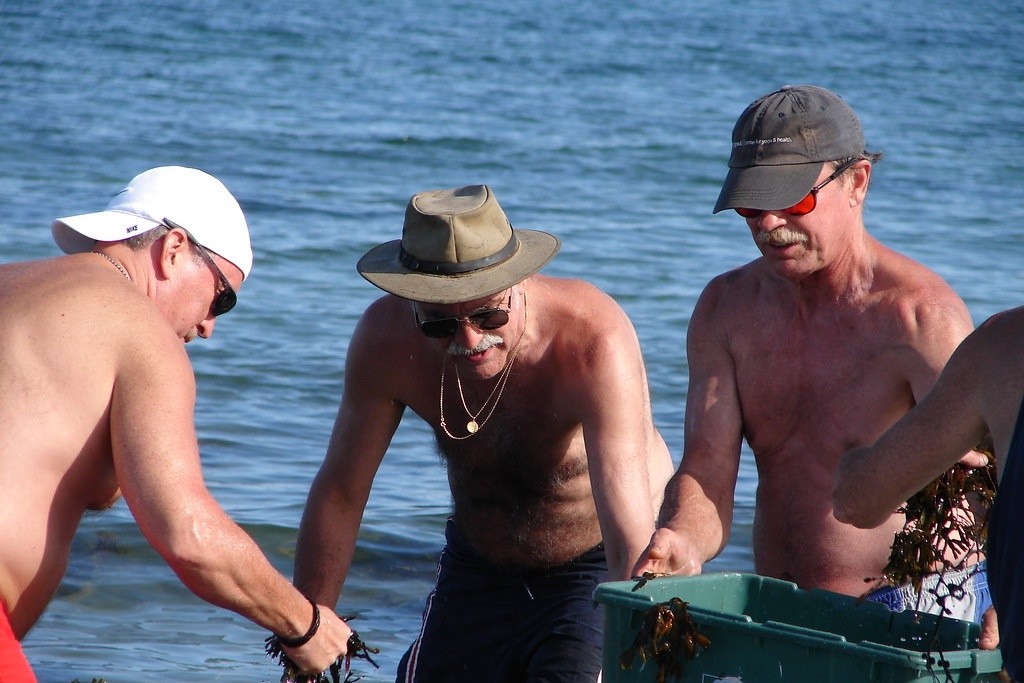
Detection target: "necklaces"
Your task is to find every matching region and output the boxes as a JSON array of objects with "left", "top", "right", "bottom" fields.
[
  {"left": 441, "top": 288, "right": 528, "bottom": 439},
  {"left": 91, "top": 250, "right": 131, "bottom": 281}
]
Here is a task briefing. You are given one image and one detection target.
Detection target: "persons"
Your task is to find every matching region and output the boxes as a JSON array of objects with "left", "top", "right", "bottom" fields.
[
  {"left": 281, "top": 186, "right": 673, "bottom": 683},
  {"left": 829, "top": 307, "right": 1024, "bottom": 683},
  {"left": 638, "top": 86, "right": 999, "bottom": 683},
  {"left": 0, "top": 165, "right": 353, "bottom": 683}
]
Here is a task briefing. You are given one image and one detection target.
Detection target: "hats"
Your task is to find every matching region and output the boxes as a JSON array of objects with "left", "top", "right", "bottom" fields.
[
  {"left": 52, "top": 166, "right": 253, "bottom": 284},
  {"left": 712, "top": 85, "right": 864, "bottom": 214},
  {"left": 357, "top": 183, "right": 560, "bottom": 305}
]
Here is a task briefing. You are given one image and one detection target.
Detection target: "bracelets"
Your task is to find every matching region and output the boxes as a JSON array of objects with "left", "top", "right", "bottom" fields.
[{"left": 278, "top": 599, "right": 320, "bottom": 648}]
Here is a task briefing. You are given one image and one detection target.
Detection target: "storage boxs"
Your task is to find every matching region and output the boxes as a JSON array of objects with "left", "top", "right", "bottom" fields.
[{"left": 591, "top": 572, "right": 1004, "bottom": 683}]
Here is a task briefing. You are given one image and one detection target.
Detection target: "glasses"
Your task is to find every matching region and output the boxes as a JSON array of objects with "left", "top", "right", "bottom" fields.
[
  {"left": 733, "top": 158, "right": 860, "bottom": 219},
  {"left": 163, "top": 217, "right": 237, "bottom": 317},
  {"left": 413, "top": 287, "right": 512, "bottom": 338}
]
